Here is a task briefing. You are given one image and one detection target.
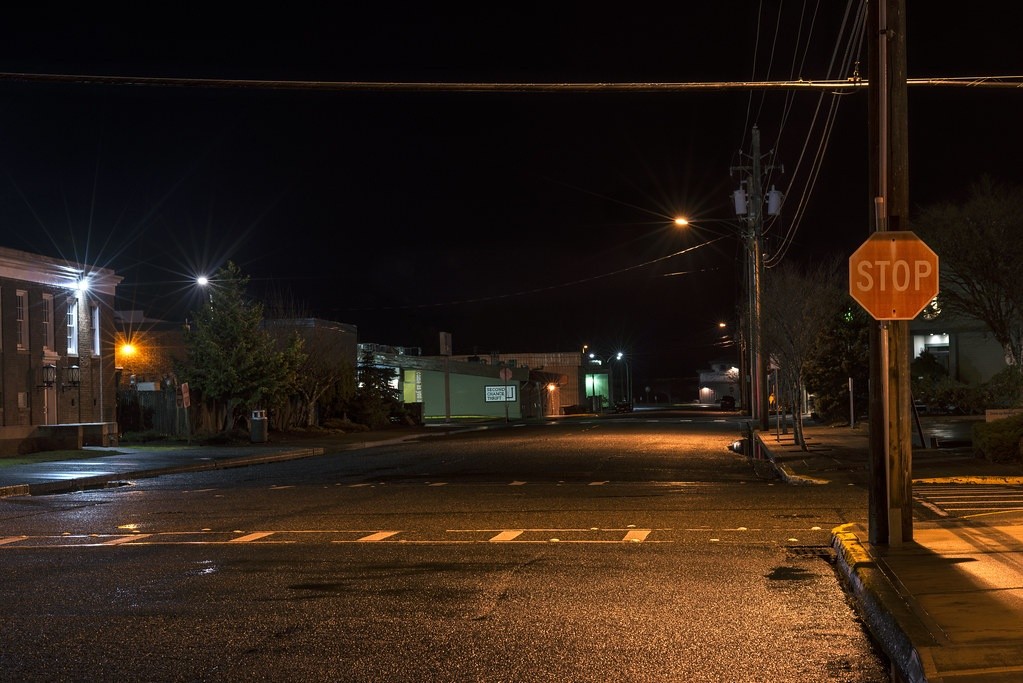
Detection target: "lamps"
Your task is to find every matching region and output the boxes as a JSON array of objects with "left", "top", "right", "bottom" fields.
[
  {"left": 34, "top": 362, "right": 56, "bottom": 388},
  {"left": 61, "top": 364, "right": 80, "bottom": 393}
]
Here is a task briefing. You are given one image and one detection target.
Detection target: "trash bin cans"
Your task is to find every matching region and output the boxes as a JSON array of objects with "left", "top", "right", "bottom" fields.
[{"left": 249, "top": 409, "right": 269, "bottom": 443}]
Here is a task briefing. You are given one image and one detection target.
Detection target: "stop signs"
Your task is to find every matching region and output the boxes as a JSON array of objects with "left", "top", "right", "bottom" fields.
[{"left": 850, "top": 230, "right": 940, "bottom": 322}]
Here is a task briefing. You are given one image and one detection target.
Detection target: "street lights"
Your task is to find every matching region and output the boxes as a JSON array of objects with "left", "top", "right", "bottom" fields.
[{"left": 674, "top": 214, "right": 760, "bottom": 420}]
